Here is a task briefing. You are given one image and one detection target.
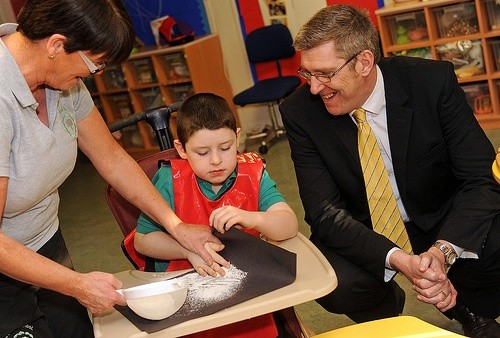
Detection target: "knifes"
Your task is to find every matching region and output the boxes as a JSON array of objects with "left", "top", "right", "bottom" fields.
[{"left": 114, "top": 274, "right": 190, "bottom": 300}]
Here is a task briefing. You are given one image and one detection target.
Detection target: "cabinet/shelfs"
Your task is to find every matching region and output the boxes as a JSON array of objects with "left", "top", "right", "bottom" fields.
[
  {"left": 90, "top": 34, "right": 240, "bottom": 152},
  {"left": 375, "top": 0, "right": 500, "bottom": 129}
]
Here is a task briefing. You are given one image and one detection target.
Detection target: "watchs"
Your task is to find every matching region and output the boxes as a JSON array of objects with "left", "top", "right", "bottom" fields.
[{"left": 432, "top": 242, "right": 457, "bottom": 267}]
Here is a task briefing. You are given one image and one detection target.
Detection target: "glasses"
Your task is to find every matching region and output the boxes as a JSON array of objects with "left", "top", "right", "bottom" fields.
[
  {"left": 297, "top": 51, "right": 364, "bottom": 83},
  {"left": 78, "top": 49, "right": 106, "bottom": 75}
]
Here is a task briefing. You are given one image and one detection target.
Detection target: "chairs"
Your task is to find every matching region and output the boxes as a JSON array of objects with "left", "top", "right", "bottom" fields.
[
  {"left": 106, "top": 148, "right": 309, "bottom": 338},
  {"left": 233, "top": 23, "right": 302, "bottom": 155}
]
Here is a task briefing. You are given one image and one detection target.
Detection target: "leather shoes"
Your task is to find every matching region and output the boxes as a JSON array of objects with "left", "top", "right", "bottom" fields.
[{"left": 456, "top": 308, "right": 500, "bottom": 338}]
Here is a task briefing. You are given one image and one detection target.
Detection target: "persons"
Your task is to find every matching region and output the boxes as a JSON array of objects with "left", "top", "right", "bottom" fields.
[
  {"left": 133, "top": 93, "right": 298, "bottom": 338},
  {"left": 280, "top": 4, "right": 500, "bottom": 338},
  {"left": 0, "top": 0, "right": 222, "bottom": 338}
]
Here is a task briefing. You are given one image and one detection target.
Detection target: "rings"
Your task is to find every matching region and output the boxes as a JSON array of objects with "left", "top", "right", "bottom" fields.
[{"left": 441, "top": 291, "right": 447, "bottom": 298}]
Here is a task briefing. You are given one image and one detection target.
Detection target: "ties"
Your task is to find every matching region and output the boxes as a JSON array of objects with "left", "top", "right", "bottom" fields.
[{"left": 353, "top": 108, "right": 413, "bottom": 273}]
